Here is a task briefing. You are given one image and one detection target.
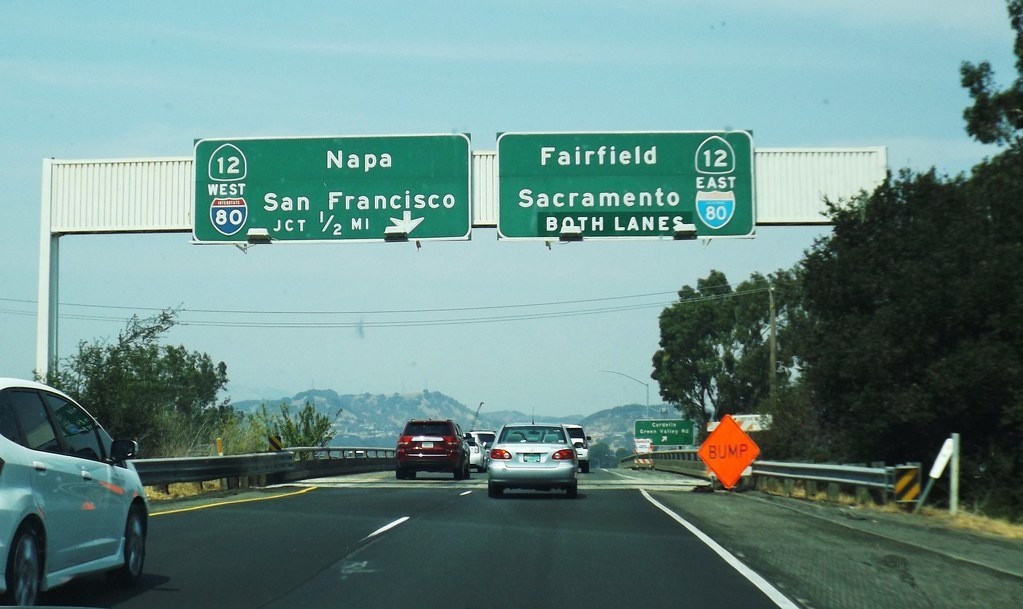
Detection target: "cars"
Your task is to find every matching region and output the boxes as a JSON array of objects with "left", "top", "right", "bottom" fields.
[
  {"left": 486, "top": 422, "right": 583, "bottom": 497},
  {"left": 461, "top": 432, "right": 490, "bottom": 470},
  {"left": 547, "top": 424, "right": 594, "bottom": 472},
  {"left": 470, "top": 428, "right": 497, "bottom": 453},
  {"left": 0, "top": 376, "right": 151, "bottom": 603}
]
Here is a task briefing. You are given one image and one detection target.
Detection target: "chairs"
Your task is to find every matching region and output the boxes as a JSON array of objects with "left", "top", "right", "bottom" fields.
[
  {"left": 505, "top": 434, "right": 524, "bottom": 443},
  {"left": 542, "top": 433, "right": 559, "bottom": 443}
]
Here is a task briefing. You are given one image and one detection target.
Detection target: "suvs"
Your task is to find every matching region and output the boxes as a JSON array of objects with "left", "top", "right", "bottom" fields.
[{"left": 397, "top": 418, "right": 472, "bottom": 481}]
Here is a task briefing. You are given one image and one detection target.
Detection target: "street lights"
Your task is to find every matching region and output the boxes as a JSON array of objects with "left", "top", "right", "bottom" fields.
[{"left": 595, "top": 370, "right": 650, "bottom": 421}]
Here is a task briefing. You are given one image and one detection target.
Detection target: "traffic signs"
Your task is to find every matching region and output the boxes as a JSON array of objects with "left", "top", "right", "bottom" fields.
[
  {"left": 193, "top": 133, "right": 474, "bottom": 244},
  {"left": 633, "top": 418, "right": 695, "bottom": 446},
  {"left": 495, "top": 127, "right": 758, "bottom": 241}
]
